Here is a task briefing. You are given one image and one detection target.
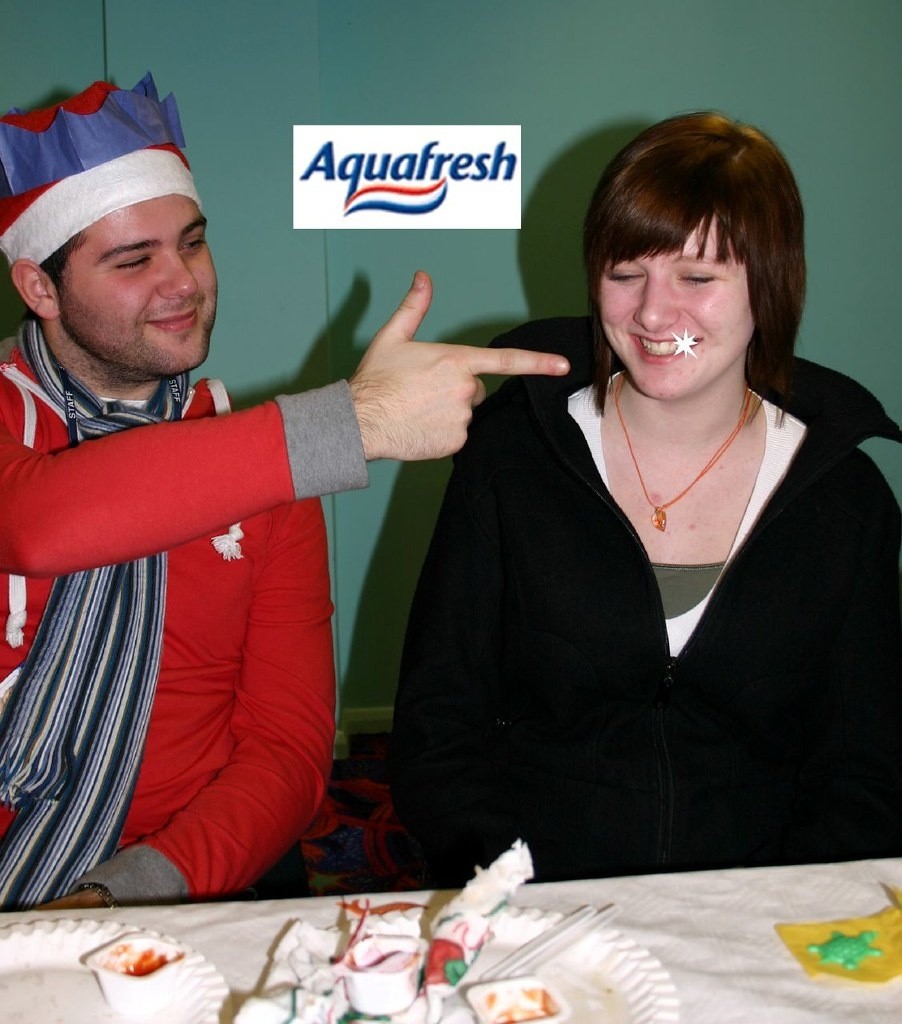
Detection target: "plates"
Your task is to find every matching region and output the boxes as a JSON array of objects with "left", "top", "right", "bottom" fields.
[
  {"left": 342, "top": 905, "right": 678, "bottom": 1024},
  {"left": 0, "top": 917, "right": 236, "bottom": 1023}
]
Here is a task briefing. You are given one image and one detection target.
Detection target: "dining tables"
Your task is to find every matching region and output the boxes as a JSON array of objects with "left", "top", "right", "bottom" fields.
[{"left": 0, "top": 857, "right": 901, "bottom": 1024}]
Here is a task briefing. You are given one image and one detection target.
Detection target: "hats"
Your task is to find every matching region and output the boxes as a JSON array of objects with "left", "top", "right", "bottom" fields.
[{"left": 0, "top": 69, "right": 202, "bottom": 267}]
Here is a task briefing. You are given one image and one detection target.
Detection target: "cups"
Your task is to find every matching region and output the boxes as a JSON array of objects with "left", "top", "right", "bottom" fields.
[
  {"left": 85, "top": 933, "right": 186, "bottom": 1013},
  {"left": 344, "top": 935, "right": 426, "bottom": 1015},
  {"left": 466, "top": 976, "right": 571, "bottom": 1024}
]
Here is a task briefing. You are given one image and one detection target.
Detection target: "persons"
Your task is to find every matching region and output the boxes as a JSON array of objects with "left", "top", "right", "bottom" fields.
[
  {"left": 0, "top": 72, "right": 573, "bottom": 922},
  {"left": 390, "top": 110, "right": 902, "bottom": 887}
]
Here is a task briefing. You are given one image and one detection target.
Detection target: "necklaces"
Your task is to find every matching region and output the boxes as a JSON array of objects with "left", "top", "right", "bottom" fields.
[{"left": 616, "top": 371, "right": 752, "bottom": 532}]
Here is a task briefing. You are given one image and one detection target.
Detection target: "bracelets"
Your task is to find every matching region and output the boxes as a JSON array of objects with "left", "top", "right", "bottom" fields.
[{"left": 79, "top": 883, "right": 118, "bottom": 908}]
